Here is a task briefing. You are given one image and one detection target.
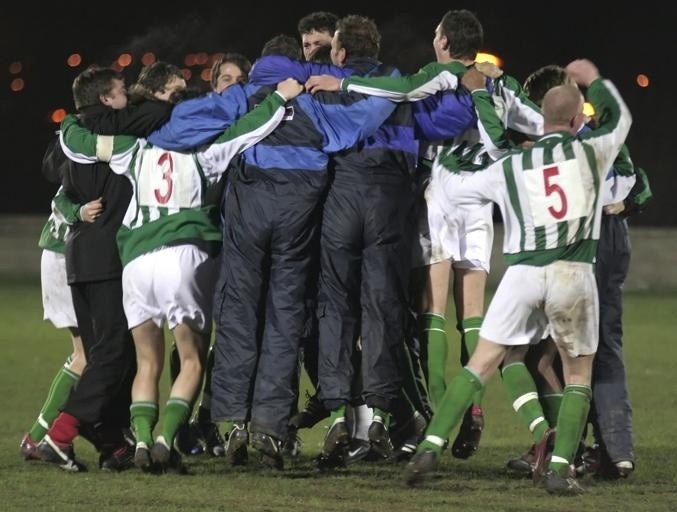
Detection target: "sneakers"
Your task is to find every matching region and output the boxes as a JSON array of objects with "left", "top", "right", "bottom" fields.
[
  {"left": 395, "top": 438, "right": 424, "bottom": 460},
  {"left": 99, "top": 442, "right": 136, "bottom": 471},
  {"left": 404, "top": 447, "right": 436, "bottom": 482},
  {"left": 319, "top": 421, "right": 350, "bottom": 467},
  {"left": 544, "top": 470, "right": 584, "bottom": 496},
  {"left": 38, "top": 435, "right": 87, "bottom": 474},
  {"left": 19, "top": 432, "right": 41, "bottom": 460},
  {"left": 191, "top": 416, "right": 225, "bottom": 456},
  {"left": 368, "top": 422, "right": 394, "bottom": 458},
  {"left": 452, "top": 414, "right": 483, "bottom": 458},
  {"left": 297, "top": 397, "right": 330, "bottom": 427},
  {"left": 151, "top": 443, "right": 183, "bottom": 474},
  {"left": 609, "top": 461, "right": 633, "bottom": 481},
  {"left": 283, "top": 439, "right": 299, "bottom": 454},
  {"left": 392, "top": 412, "right": 424, "bottom": 446},
  {"left": 508, "top": 444, "right": 536, "bottom": 471},
  {"left": 135, "top": 448, "right": 151, "bottom": 473},
  {"left": 176, "top": 425, "right": 201, "bottom": 455},
  {"left": 227, "top": 424, "right": 248, "bottom": 465},
  {"left": 252, "top": 432, "right": 283, "bottom": 470},
  {"left": 347, "top": 437, "right": 372, "bottom": 464}
]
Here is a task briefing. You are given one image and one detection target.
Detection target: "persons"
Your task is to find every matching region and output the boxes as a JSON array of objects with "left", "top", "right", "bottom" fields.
[
  {"left": 59, "top": 69, "right": 173, "bottom": 473},
  {"left": 251, "top": 18, "right": 501, "bottom": 466},
  {"left": 173, "top": 56, "right": 249, "bottom": 454},
  {"left": 59, "top": 79, "right": 304, "bottom": 470},
  {"left": 510, "top": 59, "right": 651, "bottom": 470},
  {"left": 300, "top": 13, "right": 373, "bottom": 463},
  {"left": 149, "top": 38, "right": 402, "bottom": 469},
  {"left": 21, "top": 186, "right": 103, "bottom": 467},
  {"left": 306, "top": 10, "right": 546, "bottom": 455},
  {"left": 404, "top": 59, "right": 632, "bottom": 492},
  {"left": 115, "top": 64, "right": 186, "bottom": 448}
]
